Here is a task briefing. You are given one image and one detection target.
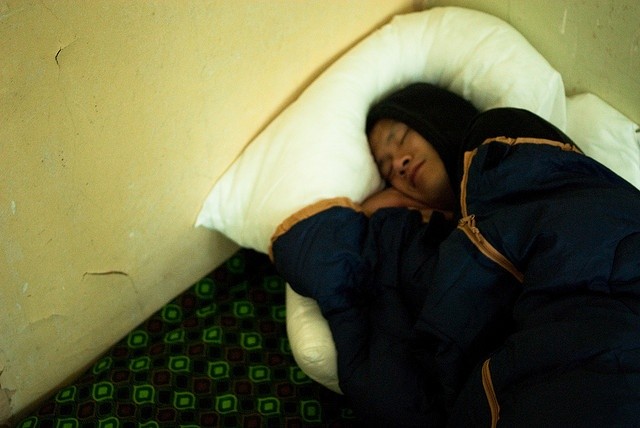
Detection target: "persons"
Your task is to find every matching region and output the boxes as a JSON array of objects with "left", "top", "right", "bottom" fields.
[{"left": 270, "top": 79, "right": 640, "bottom": 427}]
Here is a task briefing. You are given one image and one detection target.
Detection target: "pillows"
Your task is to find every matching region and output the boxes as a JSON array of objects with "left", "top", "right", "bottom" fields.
[{"left": 194, "top": 5, "right": 570, "bottom": 272}]
